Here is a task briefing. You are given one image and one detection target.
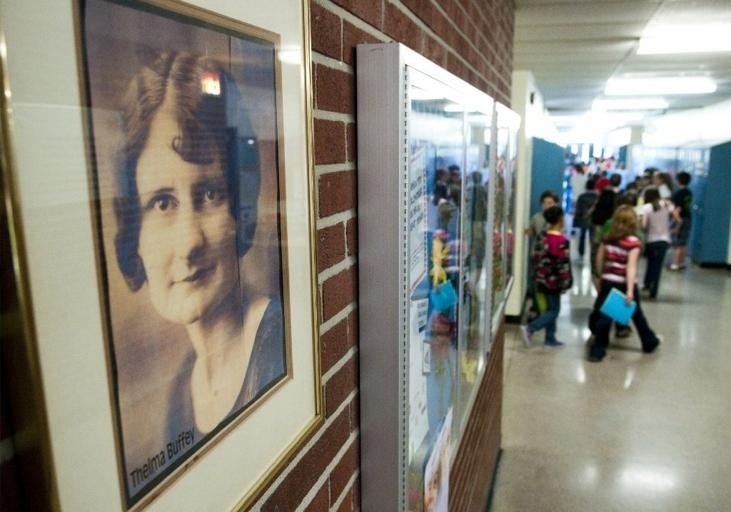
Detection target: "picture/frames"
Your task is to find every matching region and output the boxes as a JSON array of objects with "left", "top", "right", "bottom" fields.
[{"left": 0, "top": 0, "right": 324, "bottom": 512}]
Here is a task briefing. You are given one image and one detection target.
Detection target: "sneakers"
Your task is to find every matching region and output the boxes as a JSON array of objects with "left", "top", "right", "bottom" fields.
[
  {"left": 545, "top": 341, "right": 565, "bottom": 348},
  {"left": 521, "top": 324, "right": 532, "bottom": 346}
]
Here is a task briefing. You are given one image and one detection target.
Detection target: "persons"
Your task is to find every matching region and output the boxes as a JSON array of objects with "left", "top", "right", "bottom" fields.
[
  {"left": 517, "top": 155, "right": 693, "bottom": 361},
  {"left": 426, "top": 157, "right": 516, "bottom": 348},
  {"left": 112, "top": 52, "right": 286, "bottom": 470}
]
